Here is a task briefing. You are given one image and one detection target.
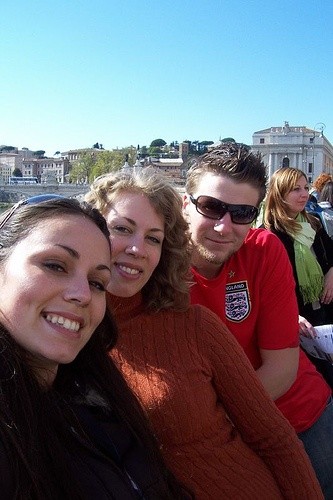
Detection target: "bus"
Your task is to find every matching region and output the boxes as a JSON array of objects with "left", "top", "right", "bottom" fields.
[{"left": 9, "top": 177, "right": 39, "bottom": 184}]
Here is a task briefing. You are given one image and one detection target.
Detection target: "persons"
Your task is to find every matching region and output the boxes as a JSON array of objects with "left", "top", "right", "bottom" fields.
[
  {"left": 0, "top": 194, "right": 197, "bottom": 500},
  {"left": 258, "top": 166, "right": 333, "bottom": 388},
  {"left": 182, "top": 143, "right": 333, "bottom": 500},
  {"left": 80, "top": 166, "right": 324, "bottom": 500}
]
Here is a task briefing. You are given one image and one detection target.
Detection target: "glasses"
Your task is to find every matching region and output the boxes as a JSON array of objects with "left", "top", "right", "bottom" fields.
[
  {"left": 0, "top": 194, "right": 102, "bottom": 231},
  {"left": 189, "top": 193, "right": 259, "bottom": 224}
]
